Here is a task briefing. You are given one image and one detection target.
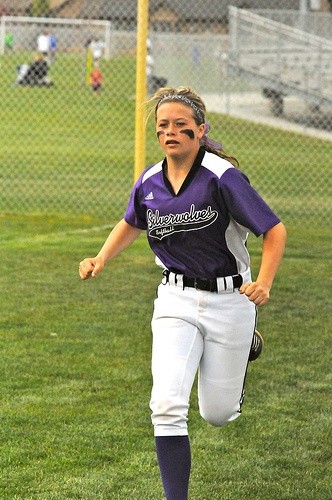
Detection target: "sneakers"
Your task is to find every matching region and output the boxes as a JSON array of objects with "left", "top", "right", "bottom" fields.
[{"left": 248, "top": 330, "right": 265, "bottom": 361}]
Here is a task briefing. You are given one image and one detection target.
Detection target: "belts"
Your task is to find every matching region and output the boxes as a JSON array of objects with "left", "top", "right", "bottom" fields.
[{"left": 164, "top": 270, "right": 243, "bottom": 292}]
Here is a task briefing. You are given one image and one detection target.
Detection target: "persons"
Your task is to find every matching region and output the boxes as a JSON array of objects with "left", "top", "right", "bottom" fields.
[
  {"left": 6, "top": 31, "right": 286, "bottom": 115},
  {"left": 79, "top": 87, "right": 286, "bottom": 500}
]
